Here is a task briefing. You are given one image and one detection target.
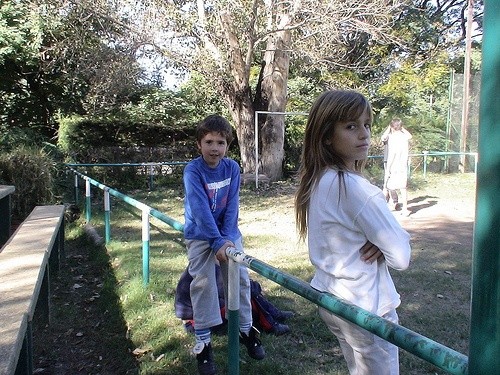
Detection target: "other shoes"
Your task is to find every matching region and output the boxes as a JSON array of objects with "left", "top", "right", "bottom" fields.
[
  {"left": 279, "top": 310, "right": 294, "bottom": 320},
  {"left": 272, "top": 322, "right": 290, "bottom": 336},
  {"left": 401, "top": 208, "right": 408, "bottom": 215}
]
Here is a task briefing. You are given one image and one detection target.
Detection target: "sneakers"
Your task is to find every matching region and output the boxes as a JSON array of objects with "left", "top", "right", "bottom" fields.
[
  {"left": 239, "top": 328, "right": 265, "bottom": 360},
  {"left": 196, "top": 340, "right": 215, "bottom": 375}
]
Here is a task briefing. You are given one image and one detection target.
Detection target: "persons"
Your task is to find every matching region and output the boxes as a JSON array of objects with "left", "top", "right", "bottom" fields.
[
  {"left": 295, "top": 90, "right": 412, "bottom": 375},
  {"left": 379, "top": 117, "right": 412, "bottom": 217},
  {"left": 173, "top": 113, "right": 295, "bottom": 375}
]
